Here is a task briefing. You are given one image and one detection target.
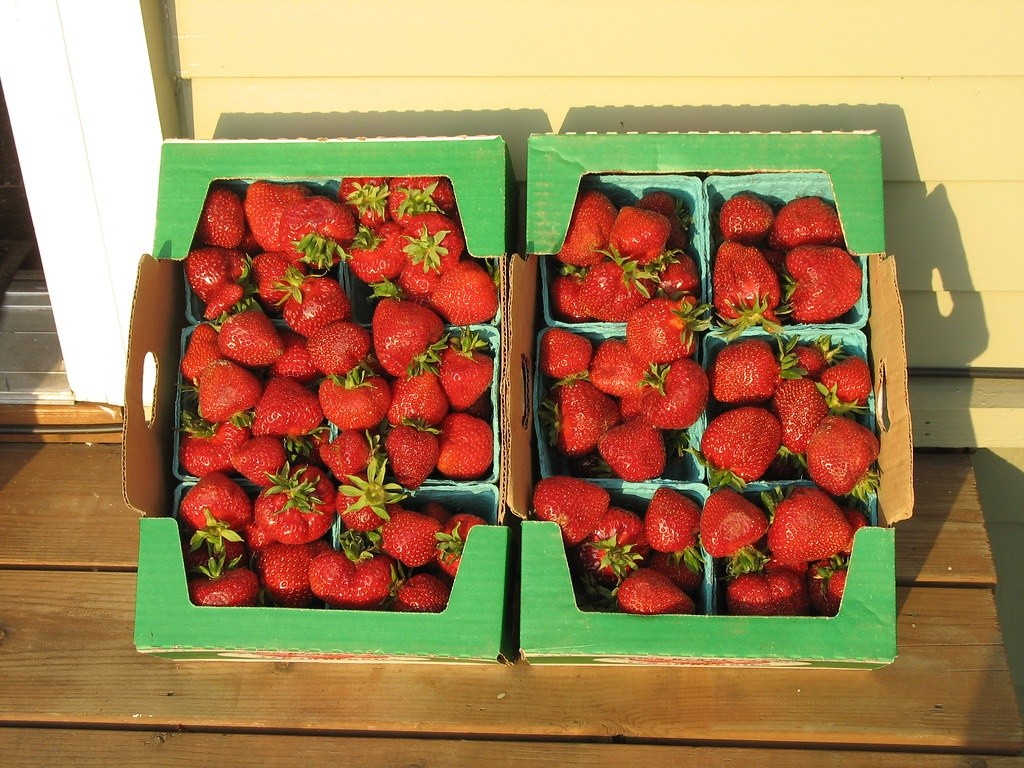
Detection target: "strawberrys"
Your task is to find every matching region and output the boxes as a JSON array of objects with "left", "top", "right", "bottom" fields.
[
  {"left": 531, "top": 184, "right": 882, "bottom": 618},
  {"left": 177, "top": 179, "right": 499, "bottom": 613}
]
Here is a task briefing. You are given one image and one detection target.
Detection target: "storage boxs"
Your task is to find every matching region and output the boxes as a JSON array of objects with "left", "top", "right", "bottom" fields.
[
  {"left": 506, "top": 128, "right": 915, "bottom": 672},
  {"left": 121, "top": 129, "right": 514, "bottom": 663}
]
